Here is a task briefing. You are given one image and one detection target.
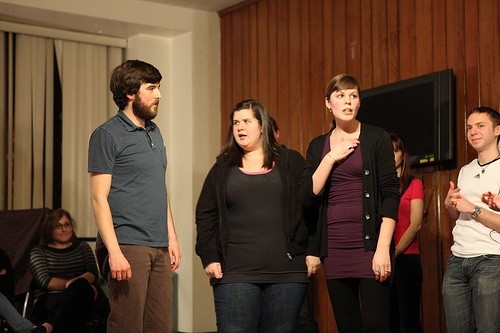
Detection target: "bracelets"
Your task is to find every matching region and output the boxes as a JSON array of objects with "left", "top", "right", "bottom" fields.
[
  {"left": 327, "top": 152, "right": 337, "bottom": 161},
  {"left": 471, "top": 205, "right": 482, "bottom": 218}
]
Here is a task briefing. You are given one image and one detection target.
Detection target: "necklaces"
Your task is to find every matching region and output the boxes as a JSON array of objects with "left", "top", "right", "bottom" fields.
[{"left": 480, "top": 153, "right": 500, "bottom": 174}]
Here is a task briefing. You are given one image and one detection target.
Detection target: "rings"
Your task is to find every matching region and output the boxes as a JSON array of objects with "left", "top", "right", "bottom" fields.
[
  {"left": 375, "top": 269, "right": 380, "bottom": 272},
  {"left": 347, "top": 145, "right": 352, "bottom": 149},
  {"left": 384, "top": 269, "right": 389, "bottom": 272}
]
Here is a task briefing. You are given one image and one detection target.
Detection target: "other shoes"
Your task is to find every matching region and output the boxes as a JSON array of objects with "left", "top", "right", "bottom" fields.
[{"left": 30, "top": 326, "right": 47, "bottom": 333}]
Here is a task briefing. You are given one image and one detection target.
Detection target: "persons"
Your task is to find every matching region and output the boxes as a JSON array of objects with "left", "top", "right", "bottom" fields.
[
  {"left": 29, "top": 208, "right": 105, "bottom": 333},
  {"left": 481, "top": 192, "right": 500, "bottom": 212},
  {"left": 0, "top": 268, "right": 53, "bottom": 333},
  {"left": 442, "top": 107, "right": 500, "bottom": 333},
  {"left": 194, "top": 98, "right": 321, "bottom": 333},
  {"left": 306, "top": 73, "right": 400, "bottom": 333},
  {"left": 88, "top": 60, "right": 182, "bottom": 333},
  {"left": 268, "top": 114, "right": 319, "bottom": 333},
  {"left": 389, "top": 131, "right": 425, "bottom": 333}
]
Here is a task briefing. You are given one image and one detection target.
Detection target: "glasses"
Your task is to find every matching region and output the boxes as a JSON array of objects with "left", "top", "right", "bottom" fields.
[{"left": 56, "top": 223, "right": 71, "bottom": 228}]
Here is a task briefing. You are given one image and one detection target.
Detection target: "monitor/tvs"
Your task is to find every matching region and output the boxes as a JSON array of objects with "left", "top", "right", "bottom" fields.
[{"left": 355, "top": 67, "right": 456, "bottom": 169}]
